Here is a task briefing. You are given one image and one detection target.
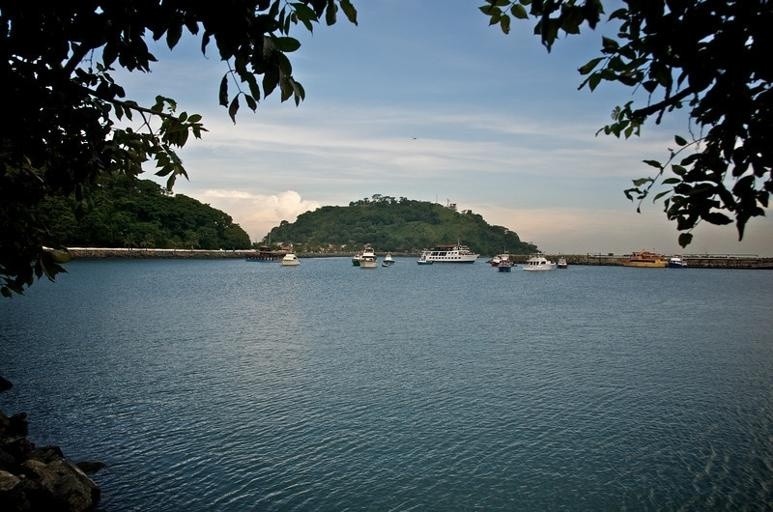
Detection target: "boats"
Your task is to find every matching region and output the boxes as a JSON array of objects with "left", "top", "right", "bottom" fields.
[
  {"left": 620, "top": 251, "right": 667, "bottom": 268},
  {"left": 352, "top": 247, "right": 377, "bottom": 268},
  {"left": 522, "top": 256, "right": 556, "bottom": 273},
  {"left": 556, "top": 256, "right": 568, "bottom": 270},
  {"left": 281, "top": 253, "right": 301, "bottom": 266},
  {"left": 416, "top": 255, "right": 435, "bottom": 265},
  {"left": 415, "top": 240, "right": 481, "bottom": 264},
  {"left": 383, "top": 252, "right": 397, "bottom": 267},
  {"left": 490, "top": 257, "right": 501, "bottom": 266},
  {"left": 668, "top": 257, "right": 688, "bottom": 267},
  {"left": 497, "top": 257, "right": 512, "bottom": 273}
]
